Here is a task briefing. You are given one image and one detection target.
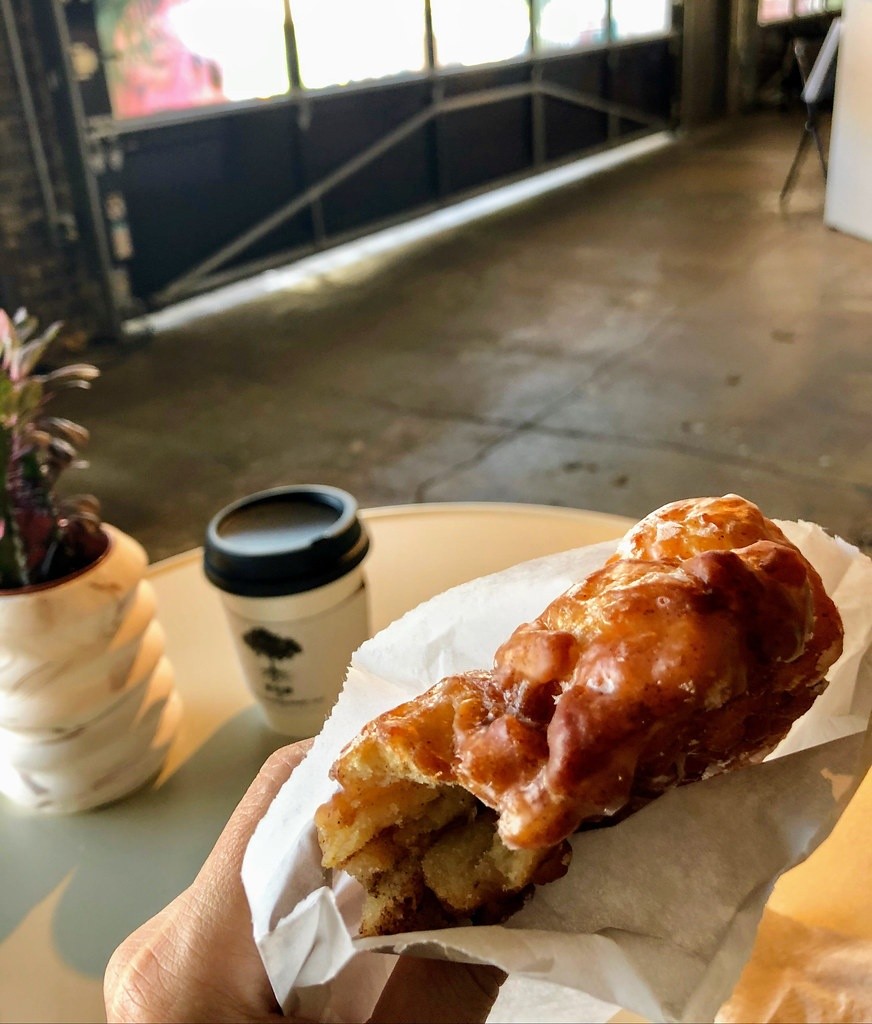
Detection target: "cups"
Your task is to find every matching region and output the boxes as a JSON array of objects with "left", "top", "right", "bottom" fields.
[{"left": 202, "top": 484, "right": 371, "bottom": 741}]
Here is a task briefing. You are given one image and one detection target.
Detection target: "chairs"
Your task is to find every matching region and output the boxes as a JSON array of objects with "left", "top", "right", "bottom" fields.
[{"left": 775, "top": 19, "right": 842, "bottom": 197}]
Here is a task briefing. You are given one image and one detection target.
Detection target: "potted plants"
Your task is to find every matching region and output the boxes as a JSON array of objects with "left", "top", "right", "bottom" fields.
[{"left": 1, "top": 308, "right": 182, "bottom": 815}]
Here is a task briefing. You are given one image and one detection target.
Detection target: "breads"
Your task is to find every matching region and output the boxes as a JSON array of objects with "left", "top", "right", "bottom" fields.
[{"left": 313, "top": 494, "right": 846, "bottom": 927}]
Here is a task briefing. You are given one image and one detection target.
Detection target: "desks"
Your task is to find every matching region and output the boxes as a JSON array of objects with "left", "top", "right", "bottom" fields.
[{"left": 0, "top": 501, "right": 871, "bottom": 1023}]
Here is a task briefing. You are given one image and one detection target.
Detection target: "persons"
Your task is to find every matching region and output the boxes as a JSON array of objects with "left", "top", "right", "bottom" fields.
[{"left": 101, "top": 732, "right": 508, "bottom": 1024}]
